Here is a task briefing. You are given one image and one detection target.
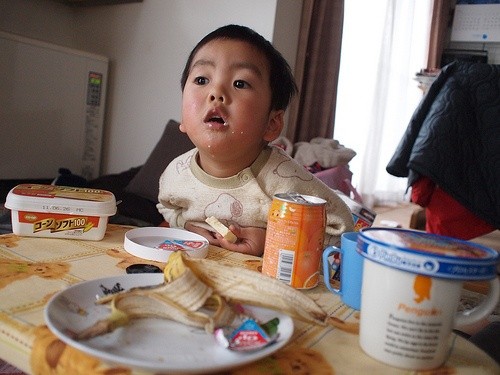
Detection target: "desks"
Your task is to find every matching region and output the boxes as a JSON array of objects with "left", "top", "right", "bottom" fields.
[{"left": 0, "top": 222, "right": 500, "bottom": 375}]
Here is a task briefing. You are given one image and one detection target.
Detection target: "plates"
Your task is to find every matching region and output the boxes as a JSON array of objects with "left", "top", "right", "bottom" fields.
[{"left": 43, "top": 273, "right": 294, "bottom": 372}]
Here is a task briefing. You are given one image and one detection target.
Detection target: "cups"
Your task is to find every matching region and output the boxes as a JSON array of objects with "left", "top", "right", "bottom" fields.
[
  {"left": 322, "top": 231, "right": 363, "bottom": 311},
  {"left": 359, "top": 258, "right": 500, "bottom": 371}
]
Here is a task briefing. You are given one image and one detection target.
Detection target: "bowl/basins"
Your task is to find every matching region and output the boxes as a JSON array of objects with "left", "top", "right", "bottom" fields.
[{"left": 4, "top": 184, "right": 116, "bottom": 241}]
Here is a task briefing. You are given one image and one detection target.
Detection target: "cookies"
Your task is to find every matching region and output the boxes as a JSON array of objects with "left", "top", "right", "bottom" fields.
[{"left": 204, "top": 216, "right": 238, "bottom": 243}]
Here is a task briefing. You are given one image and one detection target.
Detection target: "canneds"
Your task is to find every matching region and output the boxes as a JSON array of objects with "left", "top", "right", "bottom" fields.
[{"left": 261, "top": 192, "right": 327, "bottom": 290}]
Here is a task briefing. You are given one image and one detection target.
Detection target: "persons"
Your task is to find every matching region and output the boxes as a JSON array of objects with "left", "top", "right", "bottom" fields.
[{"left": 155, "top": 25, "right": 353, "bottom": 276}]
